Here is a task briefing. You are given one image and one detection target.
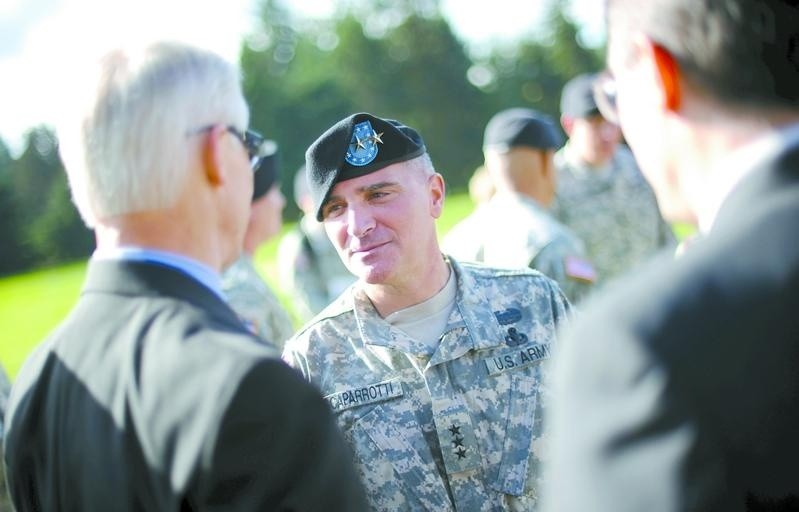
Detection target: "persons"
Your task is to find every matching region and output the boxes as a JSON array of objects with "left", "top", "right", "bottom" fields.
[
  {"left": 220, "top": 127, "right": 295, "bottom": 352},
  {"left": 537, "top": 0, "right": 799, "bottom": 512},
  {"left": 541, "top": 70, "right": 696, "bottom": 286},
  {"left": 437, "top": 105, "right": 598, "bottom": 311},
  {"left": 2, "top": 34, "right": 370, "bottom": 512},
  {"left": 274, "top": 162, "right": 359, "bottom": 324},
  {"left": 280, "top": 111, "right": 577, "bottom": 512}
]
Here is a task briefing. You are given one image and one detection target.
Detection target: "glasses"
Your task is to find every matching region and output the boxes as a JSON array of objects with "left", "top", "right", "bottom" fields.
[
  {"left": 592, "top": 77, "right": 618, "bottom": 122},
  {"left": 193, "top": 124, "right": 266, "bottom": 171}
]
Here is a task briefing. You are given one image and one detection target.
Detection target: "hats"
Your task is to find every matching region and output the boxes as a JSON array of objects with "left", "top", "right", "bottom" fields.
[
  {"left": 246, "top": 134, "right": 276, "bottom": 200},
  {"left": 559, "top": 72, "right": 614, "bottom": 117},
  {"left": 482, "top": 108, "right": 562, "bottom": 150},
  {"left": 305, "top": 112, "right": 426, "bottom": 221}
]
